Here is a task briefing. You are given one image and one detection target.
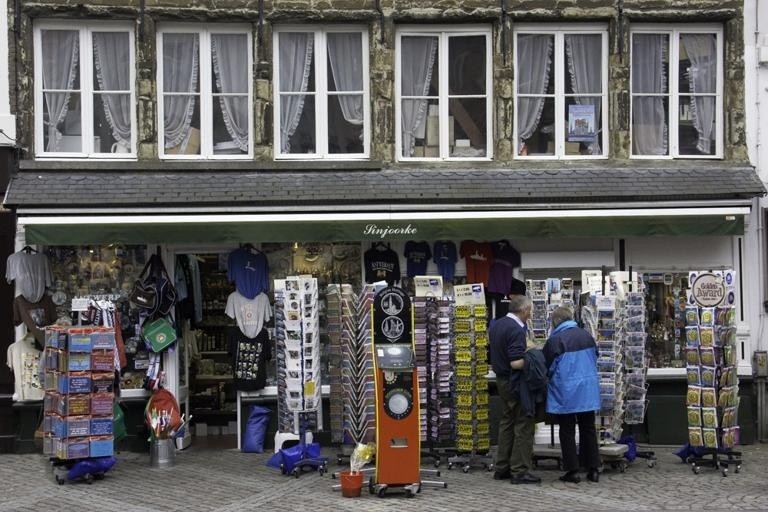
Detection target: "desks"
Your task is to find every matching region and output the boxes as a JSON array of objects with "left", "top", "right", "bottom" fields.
[{"left": 236, "top": 384, "right": 331, "bottom": 449}]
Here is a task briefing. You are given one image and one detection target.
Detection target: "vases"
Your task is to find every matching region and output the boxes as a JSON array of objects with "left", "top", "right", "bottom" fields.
[{"left": 342, "top": 471, "right": 362, "bottom": 497}]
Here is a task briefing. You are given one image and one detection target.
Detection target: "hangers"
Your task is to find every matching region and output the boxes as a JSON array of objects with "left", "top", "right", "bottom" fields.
[
  {"left": 18, "top": 243, "right": 41, "bottom": 256},
  {"left": 497, "top": 239, "right": 510, "bottom": 248},
  {"left": 370, "top": 241, "right": 389, "bottom": 250},
  {"left": 22, "top": 330, "right": 38, "bottom": 344}
]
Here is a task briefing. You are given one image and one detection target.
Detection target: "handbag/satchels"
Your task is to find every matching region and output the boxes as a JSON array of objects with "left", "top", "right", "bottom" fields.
[
  {"left": 241, "top": 403, "right": 273, "bottom": 454},
  {"left": 130, "top": 275, "right": 181, "bottom": 354}
]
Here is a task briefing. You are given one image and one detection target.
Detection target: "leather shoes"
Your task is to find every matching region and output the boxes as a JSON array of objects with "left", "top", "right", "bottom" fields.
[
  {"left": 586, "top": 470, "right": 599, "bottom": 482},
  {"left": 511, "top": 473, "right": 542, "bottom": 484},
  {"left": 494, "top": 469, "right": 512, "bottom": 480},
  {"left": 559, "top": 470, "right": 581, "bottom": 482}
]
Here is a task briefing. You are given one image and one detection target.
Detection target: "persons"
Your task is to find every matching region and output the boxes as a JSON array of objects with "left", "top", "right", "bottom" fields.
[
  {"left": 539, "top": 306, "right": 602, "bottom": 484},
  {"left": 488, "top": 295, "right": 544, "bottom": 484}
]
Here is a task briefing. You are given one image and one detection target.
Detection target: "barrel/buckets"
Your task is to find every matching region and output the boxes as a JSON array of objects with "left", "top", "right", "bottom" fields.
[
  {"left": 340, "top": 470, "right": 365, "bottom": 497},
  {"left": 150, "top": 440, "right": 176, "bottom": 469}
]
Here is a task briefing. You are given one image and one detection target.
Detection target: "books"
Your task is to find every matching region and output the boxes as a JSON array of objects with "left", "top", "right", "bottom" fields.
[
  {"left": 274, "top": 273, "right": 375, "bottom": 447},
  {"left": 526, "top": 277, "right": 574, "bottom": 349},
  {"left": 579, "top": 269, "right": 651, "bottom": 446},
  {"left": 42, "top": 325, "right": 116, "bottom": 461},
  {"left": 683, "top": 304, "right": 741, "bottom": 450},
  {"left": 412, "top": 295, "right": 453, "bottom": 443}
]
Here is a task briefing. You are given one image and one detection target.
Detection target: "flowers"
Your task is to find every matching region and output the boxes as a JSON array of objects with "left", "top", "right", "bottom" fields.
[{"left": 348, "top": 441, "right": 377, "bottom": 476}]
